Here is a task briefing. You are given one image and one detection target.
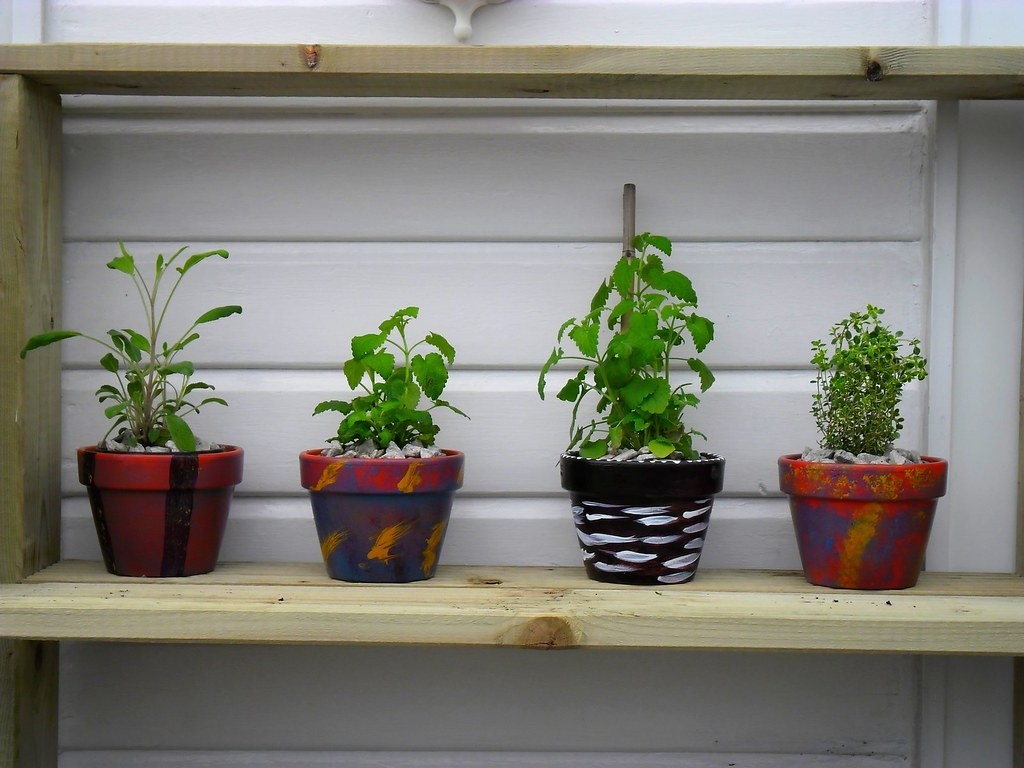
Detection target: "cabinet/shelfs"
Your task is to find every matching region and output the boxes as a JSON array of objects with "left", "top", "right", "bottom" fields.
[{"left": 0, "top": 46, "right": 1024, "bottom": 768}]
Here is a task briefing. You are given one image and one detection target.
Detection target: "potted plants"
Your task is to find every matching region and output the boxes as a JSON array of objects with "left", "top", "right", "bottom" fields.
[
  {"left": 777, "top": 307, "right": 948, "bottom": 591},
  {"left": 302, "top": 307, "right": 466, "bottom": 582},
  {"left": 538, "top": 234, "right": 726, "bottom": 585},
  {"left": 20, "top": 239, "right": 245, "bottom": 577}
]
